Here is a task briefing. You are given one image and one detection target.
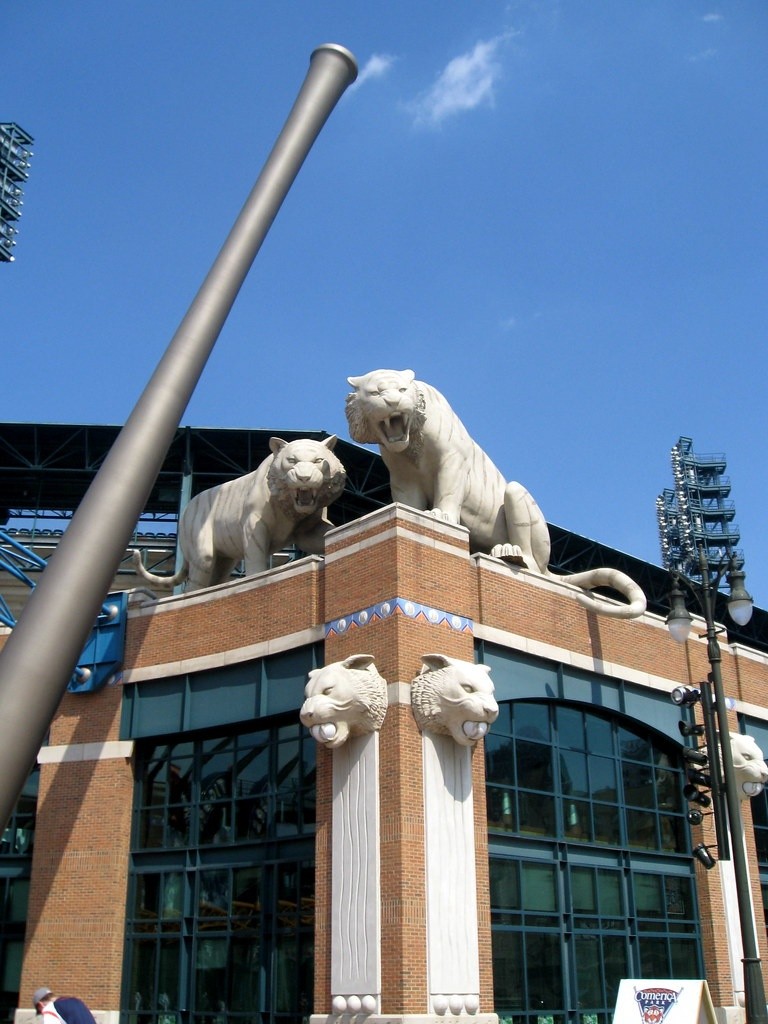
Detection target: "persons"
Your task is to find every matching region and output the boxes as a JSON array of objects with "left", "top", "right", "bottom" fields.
[{"left": 33, "top": 987, "right": 97, "bottom": 1024}]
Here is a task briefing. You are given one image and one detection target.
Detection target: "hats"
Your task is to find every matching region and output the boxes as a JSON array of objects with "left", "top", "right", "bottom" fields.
[{"left": 33, "top": 986, "right": 53, "bottom": 1015}]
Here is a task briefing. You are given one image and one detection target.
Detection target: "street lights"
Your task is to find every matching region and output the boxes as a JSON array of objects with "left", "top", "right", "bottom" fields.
[{"left": 652, "top": 435, "right": 768, "bottom": 1024}]
